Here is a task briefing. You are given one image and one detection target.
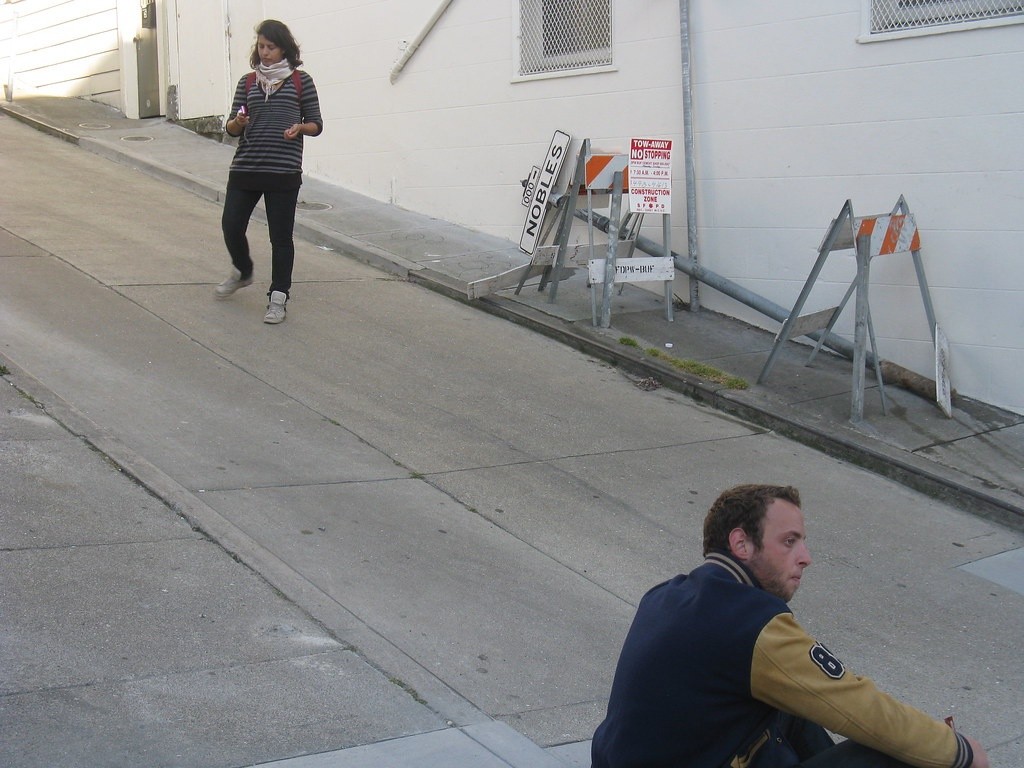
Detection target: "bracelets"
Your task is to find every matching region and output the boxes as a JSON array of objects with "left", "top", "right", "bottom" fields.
[{"left": 235, "top": 117, "right": 244, "bottom": 128}]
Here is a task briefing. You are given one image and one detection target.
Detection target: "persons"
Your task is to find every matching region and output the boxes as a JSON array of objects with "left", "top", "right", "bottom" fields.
[
  {"left": 591, "top": 484, "right": 990, "bottom": 768},
  {"left": 213, "top": 19, "right": 323, "bottom": 324}
]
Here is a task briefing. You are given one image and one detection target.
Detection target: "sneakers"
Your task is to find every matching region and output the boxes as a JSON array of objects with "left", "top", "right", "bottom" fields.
[
  {"left": 214, "top": 264, "right": 253, "bottom": 297},
  {"left": 264, "top": 290, "right": 288, "bottom": 323}
]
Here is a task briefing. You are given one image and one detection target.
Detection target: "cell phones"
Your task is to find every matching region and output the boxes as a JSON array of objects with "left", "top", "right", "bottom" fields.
[{"left": 240, "top": 105, "right": 246, "bottom": 118}]
